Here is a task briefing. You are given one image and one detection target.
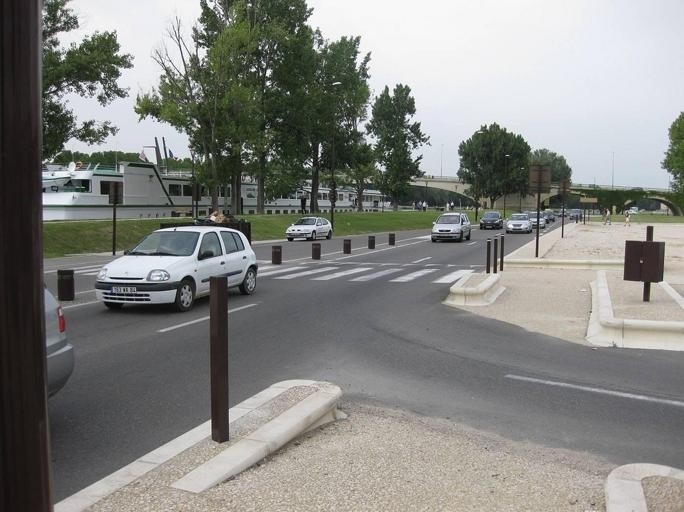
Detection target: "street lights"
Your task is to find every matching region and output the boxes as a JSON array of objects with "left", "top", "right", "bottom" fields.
[
  {"left": 504, "top": 154, "right": 510, "bottom": 218},
  {"left": 475, "top": 131, "right": 484, "bottom": 219},
  {"left": 330, "top": 81, "right": 343, "bottom": 227},
  {"left": 520, "top": 168, "right": 525, "bottom": 213}
]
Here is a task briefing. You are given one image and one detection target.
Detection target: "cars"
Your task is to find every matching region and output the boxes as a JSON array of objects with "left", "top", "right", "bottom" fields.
[
  {"left": 286, "top": 216, "right": 332, "bottom": 241},
  {"left": 94, "top": 225, "right": 258, "bottom": 312},
  {"left": 506, "top": 213, "right": 533, "bottom": 234},
  {"left": 530, "top": 211, "right": 555, "bottom": 229},
  {"left": 432, "top": 213, "right": 471, "bottom": 242},
  {"left": 479, "top": 212, "right": 503, "bottom": 230},
  {"left": 569, "top": 209, "right": 582, "bottom": 220},
  {"left": 559, "top": 211, "right": 567, "bottom": 217}
]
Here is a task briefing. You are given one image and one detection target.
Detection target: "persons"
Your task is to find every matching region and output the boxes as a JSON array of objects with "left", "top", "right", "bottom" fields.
[
  {"left": 450, "top": 200, "right": 454, "bottom": 210},
  {"left": 603, "top": 208, "right": 611, "bottom": 225},
  {"left": 412, "top": 200, "right": 416, "bottom": 210},
  {"left": 623, "top": 210, "right": 631, "bottom": 226},
  {"left": 418, "top": 200, "right": 422, "bottom": 210},
  {"left": 422, "top": 200, "right": 427, "bottom": 211}
]
